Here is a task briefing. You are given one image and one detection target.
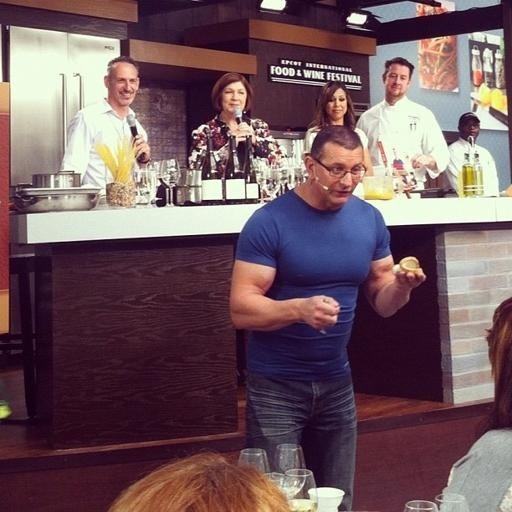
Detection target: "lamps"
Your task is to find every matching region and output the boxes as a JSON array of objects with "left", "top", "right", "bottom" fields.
[
  {"left": 343, "top": 9, "right": 383, "bottom": 36},
  {"left": 256, "top": 0, "right": 288, "bottom": 16}
]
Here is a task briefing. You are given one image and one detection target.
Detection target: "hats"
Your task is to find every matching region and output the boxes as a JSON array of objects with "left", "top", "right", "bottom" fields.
[{"left": 460, "top": 112, "right": 480, "bottom": 124}]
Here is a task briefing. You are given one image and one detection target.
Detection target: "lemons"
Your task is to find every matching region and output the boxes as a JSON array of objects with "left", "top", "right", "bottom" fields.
[{"left": 479, "top": 83, "right": 506, "bottom": 111}]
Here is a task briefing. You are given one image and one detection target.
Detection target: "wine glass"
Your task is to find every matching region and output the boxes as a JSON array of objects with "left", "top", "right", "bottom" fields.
[
  {"left": 135, "top": 155, "right": 180, "bottom": 208},
  {"left": 254, "top": 157, "right": 306, "bottom": 202}
]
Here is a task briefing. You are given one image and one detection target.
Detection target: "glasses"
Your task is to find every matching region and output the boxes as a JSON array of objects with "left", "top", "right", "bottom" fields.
[{"left": 313, "top": 158, "right": 367, "bottom": 177}]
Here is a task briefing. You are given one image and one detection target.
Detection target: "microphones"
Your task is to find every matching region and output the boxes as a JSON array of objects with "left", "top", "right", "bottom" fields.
[
  {"left": 234, "top": 106, "right": 243, "bottom": 124},
  {"left": 311, "top": 170, "right": 328, "bottom": 194},
  {"left": 127, "top": 114, "right": 146, "bottom": 160}
]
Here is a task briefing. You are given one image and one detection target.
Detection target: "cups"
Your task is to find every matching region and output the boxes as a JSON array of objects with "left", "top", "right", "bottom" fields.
[
  {"left": 308, "top": 486, "right": 345, "bottom": 512},
  {"left": 176, "top": 169, "right": 204, "bottom": 204},
  {"left": 401, "top": 495, "right": 469, "bottom": 512},
  {"left": 238, "top": 443, "right": 319, "bottom": 512}
]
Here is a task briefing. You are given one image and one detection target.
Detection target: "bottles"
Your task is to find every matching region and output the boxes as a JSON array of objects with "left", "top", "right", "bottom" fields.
[
  {"left": 222, "top": 134, "right": 247, "bottom": 204},
  {"left": 473, "top": 150, "right": 485, "bottom": 196},
  {"left": 470, "top": 44, "right": 506, "bottom": 90},
  {"left": 461, "top": 153, "right": 477, "bottom": 197},
  {"left": 242, "top": 134, "right": 260, "bottom": 205},
  {"left": 201, "top": 136, "right": 223, "bottom": 204}
]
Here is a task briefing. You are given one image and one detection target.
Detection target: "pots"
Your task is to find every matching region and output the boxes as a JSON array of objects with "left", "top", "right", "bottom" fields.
[{"left": 7, "top": 171, "right": 101, "bottom": 211}]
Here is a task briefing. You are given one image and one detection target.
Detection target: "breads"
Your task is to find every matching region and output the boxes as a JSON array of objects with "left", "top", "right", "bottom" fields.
[{"left": 399, "top": 256, "right": 422, "bottom": 273}]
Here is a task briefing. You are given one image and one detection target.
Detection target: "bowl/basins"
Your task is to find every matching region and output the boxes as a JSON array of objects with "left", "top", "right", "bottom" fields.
[{"left": 363, "top": 175, "right": 394, "bottom": 200}]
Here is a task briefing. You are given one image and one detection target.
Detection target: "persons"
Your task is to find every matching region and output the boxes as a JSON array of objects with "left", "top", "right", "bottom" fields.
[
  {"left": 186, "top": 71, "right": 289, "bottom": 203},
  {"left": 55, "top": 54, "right": 151, "bottom": 205},
  {"left": 444, "top": 110, "right": 501, "bottom": 198},
  {"left": 228, "top": 125, "right": 429, "bottom": 511},
  {"left": 304, "top": 79, "right": 375, "bottom": 186},
  {"left": 106, "top": 445, "right": 293, "bottom": 512},
  {"left": 355, "top": 56, "right": 451, "bottom": 200},
  {"left": 439, "top": 297, "right": 512, "bottom": 512}
]
